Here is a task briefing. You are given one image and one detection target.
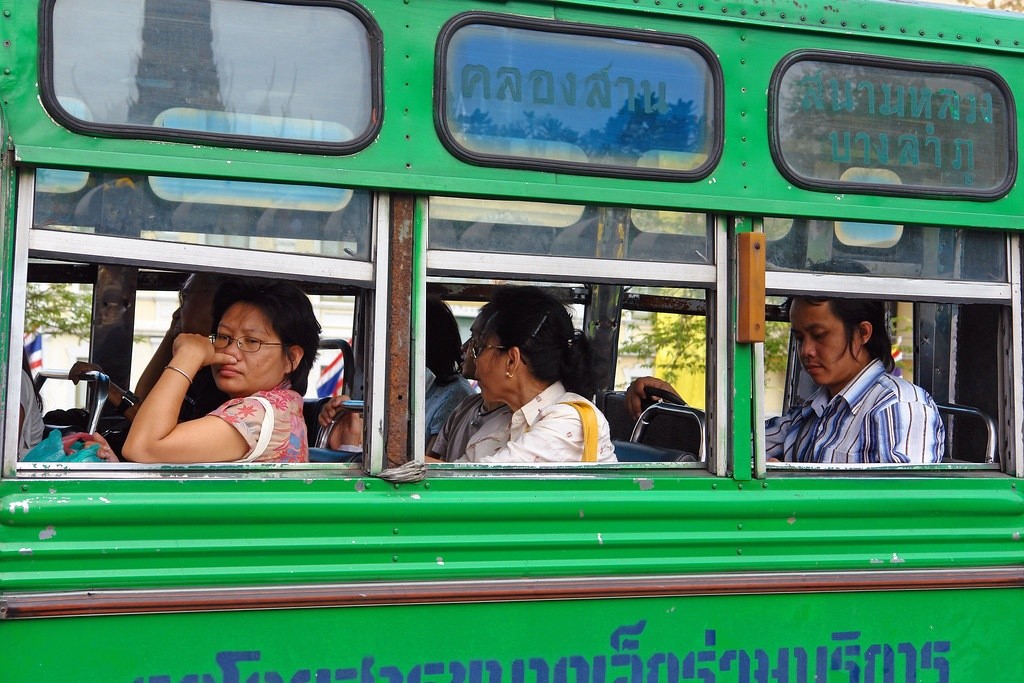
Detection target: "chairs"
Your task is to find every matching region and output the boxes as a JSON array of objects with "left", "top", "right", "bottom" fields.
[{"left": 33, "top": 337, "right": 996, "bottom": 463}]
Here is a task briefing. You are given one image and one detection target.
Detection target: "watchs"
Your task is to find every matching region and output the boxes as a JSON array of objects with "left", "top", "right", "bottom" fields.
[{"left": 117, "top": 390, "right": 139, "bottom": 414}]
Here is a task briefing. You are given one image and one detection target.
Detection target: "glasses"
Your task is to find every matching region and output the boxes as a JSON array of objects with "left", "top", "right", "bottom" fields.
[
  {"left": 469, "top": 338, "right": 528, "bottom": 366},
  {"left": 209, "top": 333, "right": 292, "bottom": 353}
]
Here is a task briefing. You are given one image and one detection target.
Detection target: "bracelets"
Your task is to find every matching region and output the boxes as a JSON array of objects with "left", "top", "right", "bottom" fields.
[{"left": 164, "top": 364, "right": 193, "bottom": 384}]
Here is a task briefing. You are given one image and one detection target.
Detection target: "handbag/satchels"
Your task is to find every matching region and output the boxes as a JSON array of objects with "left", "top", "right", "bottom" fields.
[{"left": 19, "top": 429, "right": 107, "bottom": 463}]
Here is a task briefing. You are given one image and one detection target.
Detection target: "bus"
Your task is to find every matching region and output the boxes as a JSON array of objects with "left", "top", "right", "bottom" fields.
[{"left": 2, "top": 0, "right": 1022, "bottom": 683}]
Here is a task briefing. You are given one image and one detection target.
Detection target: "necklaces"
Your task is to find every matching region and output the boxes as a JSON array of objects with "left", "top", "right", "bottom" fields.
[{"left": 469, "top": 412, "right": 483, "bottom": 426}]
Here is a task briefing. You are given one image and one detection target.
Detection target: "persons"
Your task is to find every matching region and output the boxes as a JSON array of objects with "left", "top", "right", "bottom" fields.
[
  {"left": 16, "top": 287, "right": 617, "bottom": 465},
  {"left": 624, "top": 260, "right": 946, "bottom": 466}
]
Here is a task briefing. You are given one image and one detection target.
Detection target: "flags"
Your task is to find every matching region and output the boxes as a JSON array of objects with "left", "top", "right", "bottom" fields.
[
  {"left": 22, "top": 330, "right": 43, "bottom": 378},
  {"left": 317, "top": 338, "right": 353, "bottom": 400}
]
